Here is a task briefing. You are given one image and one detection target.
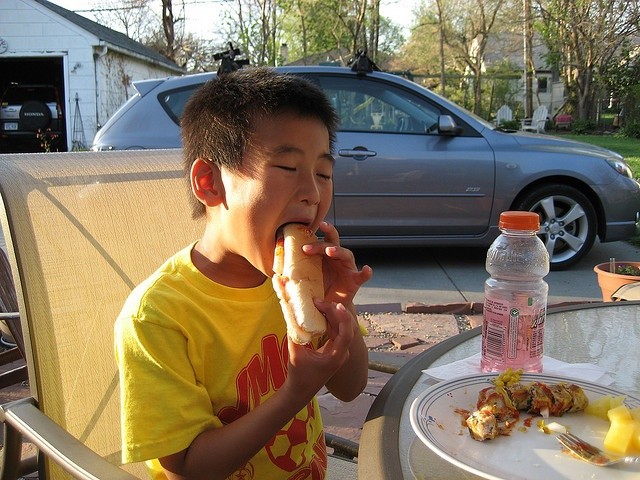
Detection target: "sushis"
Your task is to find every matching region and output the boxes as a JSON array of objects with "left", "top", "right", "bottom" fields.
[{"left": 466, "top": 383, "right": 589, "bottom": 442}]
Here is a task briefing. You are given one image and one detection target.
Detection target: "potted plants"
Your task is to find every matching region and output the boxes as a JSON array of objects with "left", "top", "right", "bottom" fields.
[{"left": 594, "top": 259, "right": 640, "bottom": 301}]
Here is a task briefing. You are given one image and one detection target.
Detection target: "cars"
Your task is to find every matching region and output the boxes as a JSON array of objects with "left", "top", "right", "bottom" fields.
[{"left": 92, "top": 65, "right": 640, "bottom": 270}]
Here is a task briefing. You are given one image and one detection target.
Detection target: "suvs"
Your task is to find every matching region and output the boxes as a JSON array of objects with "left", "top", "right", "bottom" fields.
[{"left": 0, "top": 84, "right": 64, "bottom": 147}]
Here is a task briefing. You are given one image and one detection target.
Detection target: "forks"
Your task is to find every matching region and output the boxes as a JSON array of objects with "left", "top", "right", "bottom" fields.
[{"left": 556, "top": 432, "right": 640, "bottom": 467}]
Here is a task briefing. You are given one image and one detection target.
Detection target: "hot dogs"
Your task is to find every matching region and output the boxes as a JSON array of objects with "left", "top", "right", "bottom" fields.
[{"left": 272, "top": 223, "right": 328, "bottom": 346}]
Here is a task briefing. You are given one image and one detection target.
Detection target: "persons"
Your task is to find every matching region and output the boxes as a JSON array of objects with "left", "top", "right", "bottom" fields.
[{"left": 113, "top": 67, "right": 373, "bottom": 480}]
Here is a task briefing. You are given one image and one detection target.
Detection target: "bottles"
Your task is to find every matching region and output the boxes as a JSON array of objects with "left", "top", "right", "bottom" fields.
[{"left": 480, "top": 210, "right": 550, "bottom": 374}]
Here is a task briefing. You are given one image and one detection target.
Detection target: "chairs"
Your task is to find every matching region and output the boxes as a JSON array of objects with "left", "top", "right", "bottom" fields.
[
  {"left": 493, "top": 104, "right": 512, "bottom": 129},
  {"left": 522, "top": 104, "right": 548, "bottom": 133},
  {"left": 0, "top": 148, "right": 363, "bottom": 480},
  {"left": 0, "top": 248, "right": 29, "bottom": 389}
]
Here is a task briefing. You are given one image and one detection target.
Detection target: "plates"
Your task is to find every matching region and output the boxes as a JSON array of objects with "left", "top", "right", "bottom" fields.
[
  {"left": 408, "top": 434, "right": 489, "bottom": 480},
  {"left": 408, "top": 372, "right": 640, "bottom": 480}
]
